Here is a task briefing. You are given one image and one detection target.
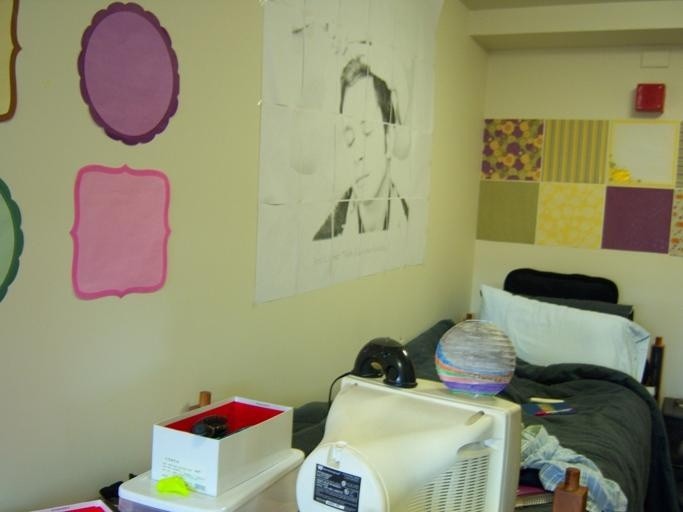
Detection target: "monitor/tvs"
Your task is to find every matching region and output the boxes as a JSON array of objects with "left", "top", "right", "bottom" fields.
[{"left": 296, "top": 374, "right": 521, "bottom": 512}]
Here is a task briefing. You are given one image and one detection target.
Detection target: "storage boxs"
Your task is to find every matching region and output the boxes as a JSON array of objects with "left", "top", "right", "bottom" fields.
[{"left": 152, "top": 397, "right": 293, "bottom": 494}]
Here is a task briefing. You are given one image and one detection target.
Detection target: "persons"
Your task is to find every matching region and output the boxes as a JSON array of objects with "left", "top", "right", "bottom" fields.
[{"left": 311, "top": 52, "right": 409, "bottom": 243}]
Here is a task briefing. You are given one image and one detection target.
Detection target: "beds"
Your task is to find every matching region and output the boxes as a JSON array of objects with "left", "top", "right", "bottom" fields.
[{"left": 290, "top": 268, "right": 682, "bottom": 510}]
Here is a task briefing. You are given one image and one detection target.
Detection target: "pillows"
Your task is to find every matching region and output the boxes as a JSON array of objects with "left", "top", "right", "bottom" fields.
[{"left": 476, "top": 269, "right": 651, "bottom": 384}]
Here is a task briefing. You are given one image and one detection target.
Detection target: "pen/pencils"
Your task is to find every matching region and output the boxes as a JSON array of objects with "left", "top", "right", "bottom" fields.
[
  {"left": 530, "top": 398, "right": 564, "bottom": 403},
  {"left": 535, "top": 408, "right": 576, "bottom": 416}
]
